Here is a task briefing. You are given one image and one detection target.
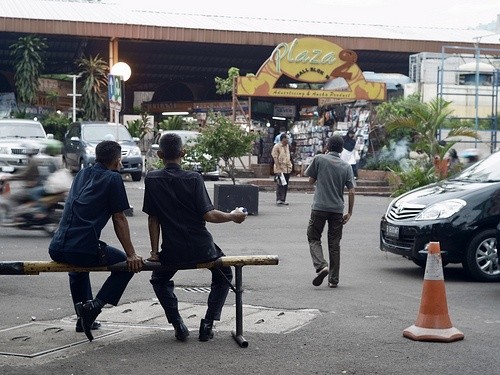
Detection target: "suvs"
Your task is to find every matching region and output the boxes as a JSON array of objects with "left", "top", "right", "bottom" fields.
[{"left": 0, "top": 118, "right": 55, "bottom": 175}]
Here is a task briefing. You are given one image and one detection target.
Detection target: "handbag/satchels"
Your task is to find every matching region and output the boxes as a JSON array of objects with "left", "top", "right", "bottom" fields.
[{"left": 275, "top": 172, "right": 287, "bottom": 186}]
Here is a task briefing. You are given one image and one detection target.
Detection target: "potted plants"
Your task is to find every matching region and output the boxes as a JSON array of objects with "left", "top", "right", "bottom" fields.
[{"left": 183, "top": 108, "right": 262, "bottom": 215}]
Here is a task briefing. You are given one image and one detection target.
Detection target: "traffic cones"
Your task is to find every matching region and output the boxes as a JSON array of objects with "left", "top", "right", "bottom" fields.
[{"left": 403, "top": 241, "right": 466, "bottom": 343}]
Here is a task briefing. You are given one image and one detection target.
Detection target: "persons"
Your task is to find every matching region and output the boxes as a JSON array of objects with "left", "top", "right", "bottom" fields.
[
  {"left": 274, "top": 127, "right": 291, "bottom": 145},
  {"left": 343, "top": 131, "right": 358, "bottom": 180},
  {"left": 435, "top": 148, "right": 460, "bottom": 177},
  {"left": 270, "top": 134, "right": 292, "bottom": 206},
  {"left": 303, "top": 135, "right": 354, "bottom": 287},
  {"left": 142, "top": 133, "right": 248, "bottom": 341},
  {"left": 0, "top": 143, "right": 44, "bottom": 205},
  {"left": 48, "top": 140, "right": 145, "bottom": 343}
]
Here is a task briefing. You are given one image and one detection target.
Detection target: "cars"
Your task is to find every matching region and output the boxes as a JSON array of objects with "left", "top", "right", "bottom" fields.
[
  {"left": 378, "top": 151, "right": 500, "bottom": 281},
  {"left": 144, "top": 130, "right": 221, "bottom": 182},
  {"left": 62, "top": 121, "right": 144, "bottom": 182}
]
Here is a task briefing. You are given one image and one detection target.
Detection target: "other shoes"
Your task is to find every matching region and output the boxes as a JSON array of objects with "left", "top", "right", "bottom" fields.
[
  {"left": 313, "top": 266, "right": 329, "bottom": 286},
  {"left": 276, "top": 199, "right": 289, "bottom": 205},
  {"left": 76, "top": 316, "right": 101, "bottom": 332},
  {"left": 199, "top": 319, "right": 214, "bottom": 341},
  {"left": 171, "top": 317, "right": 190, "bottom": 340},
  {"left": 75, "top": 300, "right": 102, "bottom": 343},
  {"left": 328, "top": 282, "right": 337, "bottom": 288}
]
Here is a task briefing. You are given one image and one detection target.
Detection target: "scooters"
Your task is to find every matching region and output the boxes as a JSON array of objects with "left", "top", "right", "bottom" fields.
[{"left": 0, "top": 169, "right": 75, "bottom": 238}]
